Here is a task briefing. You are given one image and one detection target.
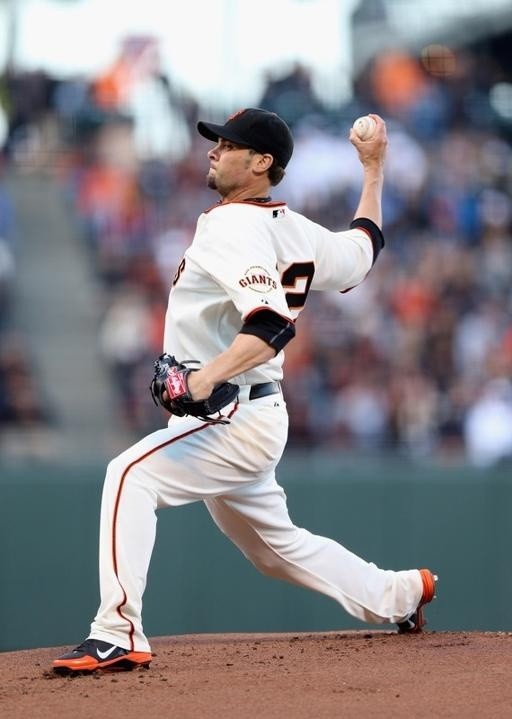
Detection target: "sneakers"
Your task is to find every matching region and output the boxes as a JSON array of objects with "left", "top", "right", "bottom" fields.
[
  {"left": 397, "top": 568, "right": 439, "bottom": 633},
  {"left": 52, "top": 639, "right": 152, "bottom": 674}
]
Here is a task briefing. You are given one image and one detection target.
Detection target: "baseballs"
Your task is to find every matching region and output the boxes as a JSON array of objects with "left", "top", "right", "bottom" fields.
[{"left": 352, "top": 116, "right": 376, "bottom": 140}]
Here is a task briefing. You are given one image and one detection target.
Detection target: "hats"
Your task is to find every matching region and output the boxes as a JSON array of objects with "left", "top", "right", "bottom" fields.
[{"left": 197, "top": 107, "right": 294, "bottom": 168}]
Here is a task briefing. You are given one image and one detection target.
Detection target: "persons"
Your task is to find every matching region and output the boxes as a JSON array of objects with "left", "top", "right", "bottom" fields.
[
  {"left": 52, "top": 107, "right": 437, "bottom": 677},
  {"left": 0, "top": 0, "right": 512, "bottom": 470}
]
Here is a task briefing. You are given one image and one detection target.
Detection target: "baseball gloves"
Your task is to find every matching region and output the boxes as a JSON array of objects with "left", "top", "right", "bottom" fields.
[{"left": 151, "top": 353, "right": 239, "bottom": 416}]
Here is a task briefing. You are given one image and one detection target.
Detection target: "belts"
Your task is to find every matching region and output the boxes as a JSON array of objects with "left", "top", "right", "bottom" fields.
[{"left": 248, "top": 380, "right": 283, "bottom": 403}]
